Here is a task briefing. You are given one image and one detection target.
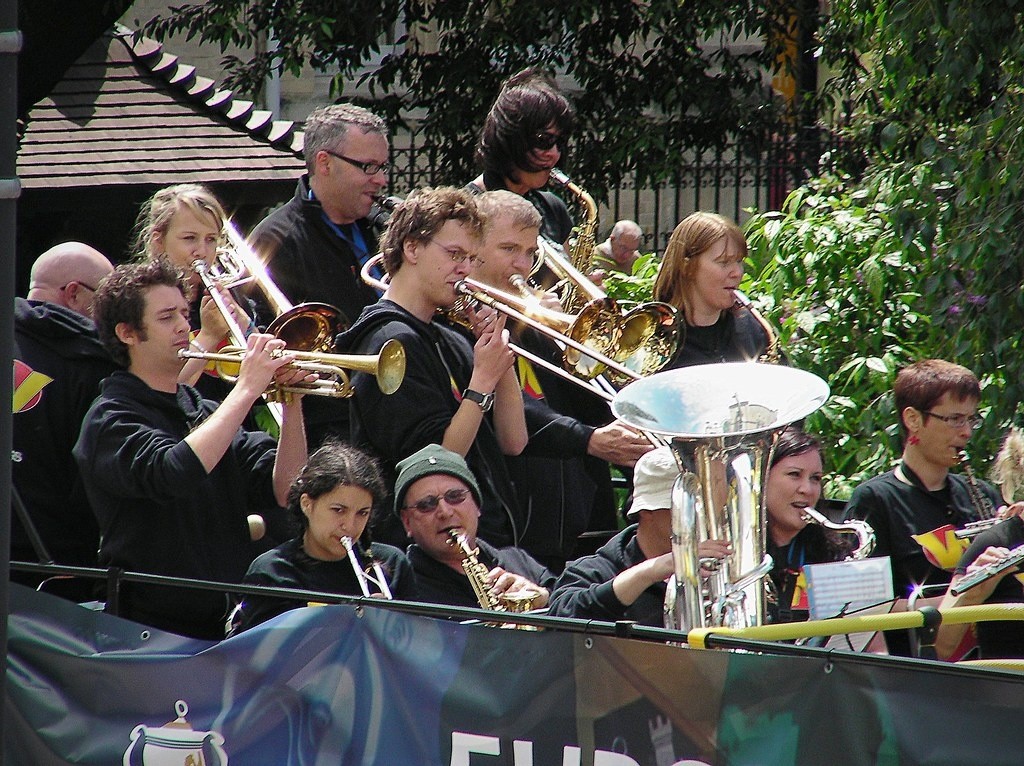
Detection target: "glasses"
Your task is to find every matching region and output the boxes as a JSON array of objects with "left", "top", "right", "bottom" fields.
[
  {"left": 429, "top": 239, "right": 486, "bottom": 268},
  {"left": 534, "top": 131, "right": 568, "bottom": 152},
  {"left": 407, "top": 488, "right": 471, "bottom": 514},
  {"left": 919, "top": 409, "right": 985, "bottom": 429},
  {"left": 320, "top": 149, "right": 389, "bottom": 175},
  {"left": 59, "top": 280, "right": 97, "bottom": 294}
]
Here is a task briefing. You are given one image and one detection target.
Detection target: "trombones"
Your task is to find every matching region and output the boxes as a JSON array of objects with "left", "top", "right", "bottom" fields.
[
  {"left": 360, "top": 248, "right": 643, "bottom": 402},
  {"left": 192, "top": 217, "right": 353, "bottom": 430},
  {"left": 509, "top": 234, "right": 683, "bottom": 388}
]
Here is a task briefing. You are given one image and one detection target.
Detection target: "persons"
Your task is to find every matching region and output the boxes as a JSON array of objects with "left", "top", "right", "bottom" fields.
[
  {"left": 434, "top": 189, "right": 656, "bottom": 574},
  {"left": 649, "top": 211, "right": 805, "bottom": 482},
  {"left": 136, "top": 185, "right": 260, "bottom": 431},
  {"left": 73, "top": 261, "right": 318, "bottom": 642},
  {"left": 761, "top": 429, "right": 891, "bottom": 646},
  {"left": 16, "top": 240, "right": 117, "bottom": 602},
  {"left": 386, "top": 442, "right": 559, "bottom": 631},
  {"left": 246, "top": 101, "right": 405, "bottom": 453},
  {"left": 237, "top": 443, "right": 417, "bottom": 630},
  {"left": 843, "top": 358, "right": 1007, "bottom": 665},
  {"left": 935, "top": 429, "right": 1024, "bottom": 663},
  {"left": 548, "top": 446, "right": 734, "bottom": 627},
  {"left": 591, "top": 221, "right": 646, "bottom": 280},
  {"left": 480, "top": 69, "right": 606, "bottom": 311},
  {"left": 333, "top": 183, "right": 547, "bottom": 550}
]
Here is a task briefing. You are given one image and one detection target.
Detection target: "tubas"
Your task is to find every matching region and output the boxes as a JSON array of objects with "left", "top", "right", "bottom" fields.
[{"left": 609, "top": 362, "right": 878, "bottom": 643}]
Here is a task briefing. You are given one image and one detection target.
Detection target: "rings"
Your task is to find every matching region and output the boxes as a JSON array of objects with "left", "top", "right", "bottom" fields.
[
  {"left": 263, "top": 347, "right": 275, "bottom": 356},
  {"left": 485, "top": 317, "right": 492, "bottom": 324}
]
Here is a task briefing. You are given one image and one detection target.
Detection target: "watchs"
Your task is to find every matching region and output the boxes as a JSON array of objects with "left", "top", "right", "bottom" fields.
[{"left": 461, "top": 388, "right": 497, "bottom": 413}]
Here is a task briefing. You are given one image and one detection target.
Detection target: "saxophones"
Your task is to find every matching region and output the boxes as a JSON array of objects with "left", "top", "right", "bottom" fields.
[
  {"left": 443, "top": 526, "right": 540, "bottom": 630},
  {"left": 800, "top": 505, "right": 878, "bottom": 560},
  {"left": 731, "top": 287, "right": 784, "bottom": 365},
  {"left": 546, "top": 165, "right": 600, "bottom": 313},
  {"left": 955, "top": 441, "right": 991, "bottom": 519},
  {"left": 371, "top": 192, "right": 479, "bottom": 322}
]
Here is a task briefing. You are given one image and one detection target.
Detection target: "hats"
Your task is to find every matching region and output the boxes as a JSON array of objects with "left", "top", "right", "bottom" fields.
[
  {"left": 626, "top": 444, "right": 680, "bottom": 519},
  {"left": 392, "top": 443, "right": 482, "bottom": 518}
]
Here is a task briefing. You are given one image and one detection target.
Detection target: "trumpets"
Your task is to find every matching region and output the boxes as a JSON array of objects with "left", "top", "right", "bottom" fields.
[
  {"left": 338, "top": 532, "right": 390, "bottom": 598},
  {"left": 174, "top": 339, "right": 405, "bottom": 398}
]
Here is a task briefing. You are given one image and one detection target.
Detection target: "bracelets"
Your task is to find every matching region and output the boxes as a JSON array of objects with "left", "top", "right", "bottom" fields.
[{"left": 192, "top": 341, "right": 217, "bottom": 364}]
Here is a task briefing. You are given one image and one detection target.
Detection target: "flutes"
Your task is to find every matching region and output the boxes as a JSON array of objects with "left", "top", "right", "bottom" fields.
[
  {"left": 952, "top": 547, "right": 1024, "bottom": 596},
  {"left": 955, "top": 513, "right": 1008, "bottom": 540}
]
